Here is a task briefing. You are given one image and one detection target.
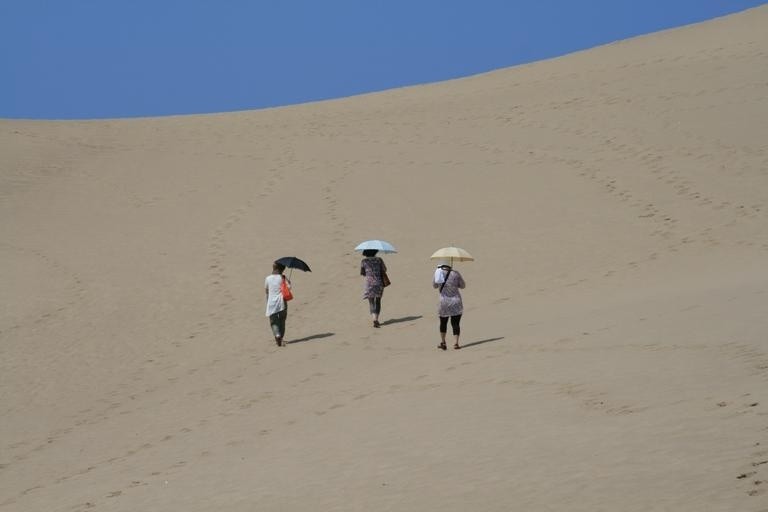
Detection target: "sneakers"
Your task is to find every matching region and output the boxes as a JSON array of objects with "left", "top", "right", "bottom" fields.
[
  {"left": 373, "top": 320, "right": 381, "bottom": 327},
  {"left": 274, "top": 336, "right": 283, "bottom": 345}
]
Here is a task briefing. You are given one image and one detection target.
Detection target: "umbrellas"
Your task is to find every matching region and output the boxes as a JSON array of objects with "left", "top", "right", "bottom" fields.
[
  {"left": 276, "top": 256, "right": 311, "bottom": 283},
  {"left": 430, "top": 244, "right": 475, "bottom": 272},
  {"left": 355, "top": 240, "right": 398, "bottom": 255}
]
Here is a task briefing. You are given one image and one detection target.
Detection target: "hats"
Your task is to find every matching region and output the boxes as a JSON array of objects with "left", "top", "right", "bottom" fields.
[{"left": 437, "top": 260, "right": 450, "bottom": 267}]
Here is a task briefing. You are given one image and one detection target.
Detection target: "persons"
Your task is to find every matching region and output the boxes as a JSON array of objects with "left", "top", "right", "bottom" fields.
[
  {"left": 265, "top": 263, "right": 292, "bottom": 347},
  {"left": 431, "top": 259, "right": 465, "bottom": 348},
  {"left": 359, "top": 248, "right": 386, "bottom": 328}
]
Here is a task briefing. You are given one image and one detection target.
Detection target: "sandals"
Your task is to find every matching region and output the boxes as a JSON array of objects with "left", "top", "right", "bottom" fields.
[
  {"left": 453, "top": 343, "right": 459, "bottom": 349},
  {"left": 437, "top": 342, "right": 447, "bottom": 349}
]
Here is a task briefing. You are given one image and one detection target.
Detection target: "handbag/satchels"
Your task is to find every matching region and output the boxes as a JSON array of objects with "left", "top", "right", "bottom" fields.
[
  {"left": 380, "top": 264, "right": 391, "bottom": 287},
  {"left": 280, "top": 275, "right": 293, "bottom": 300}
]
